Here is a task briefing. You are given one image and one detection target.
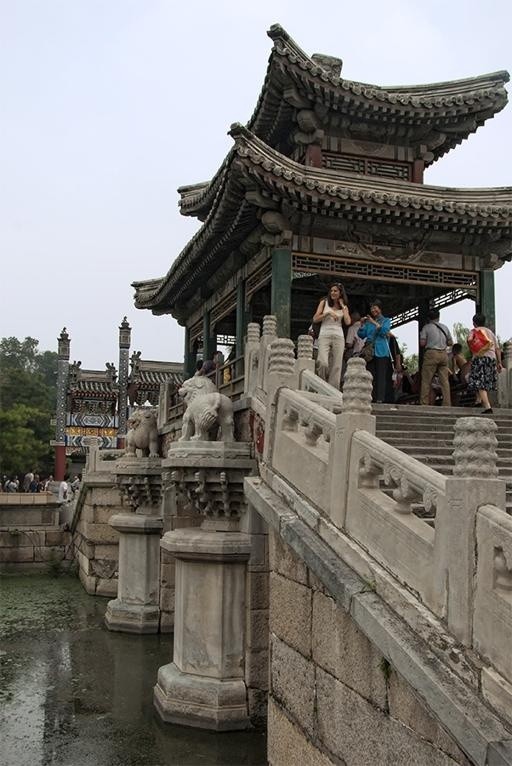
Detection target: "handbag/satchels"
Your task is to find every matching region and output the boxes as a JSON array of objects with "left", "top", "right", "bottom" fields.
[
  {"left": 361, "top": 344, "right": 374, "bottom": 361},
  {"left": 468, "top": 329, "right": 493, "bottom": 356},
  {"left": 312, "top": 299, "right": 325, "bottom": 338}
]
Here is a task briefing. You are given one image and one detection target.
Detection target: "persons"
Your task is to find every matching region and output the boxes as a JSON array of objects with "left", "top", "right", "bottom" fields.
[
  {"left": 0, "top": 468, "right": 82, "bottom": 503},
  {"left": 308, "top": 282, "right": 503, "bottom": 414},
  {"left": 193, "top": 344, "right": 236, "bottom": 384}
]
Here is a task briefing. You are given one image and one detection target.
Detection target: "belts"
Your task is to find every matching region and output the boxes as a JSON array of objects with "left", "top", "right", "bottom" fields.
[{"left": 427, "top": 348, "right": 446, "bottom": 352}]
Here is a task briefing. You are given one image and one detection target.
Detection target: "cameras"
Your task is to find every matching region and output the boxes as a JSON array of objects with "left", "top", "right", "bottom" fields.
[{"left": 363, "top": 316, "right": 369, "bottom": 320}]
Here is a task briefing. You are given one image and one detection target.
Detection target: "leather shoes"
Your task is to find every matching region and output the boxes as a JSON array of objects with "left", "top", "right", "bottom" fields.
[
  {"left": 482, "top": 408, "right": 493, "bottom": 413},
  {"left": 472, "top": 402, "right": 482, "bottom": 407}
]
